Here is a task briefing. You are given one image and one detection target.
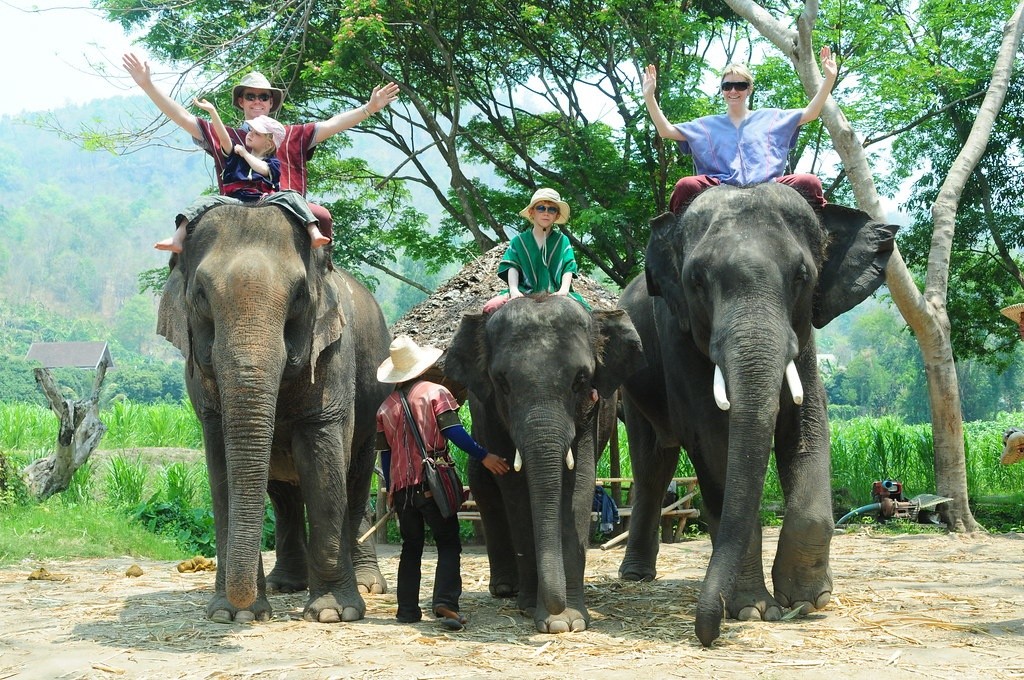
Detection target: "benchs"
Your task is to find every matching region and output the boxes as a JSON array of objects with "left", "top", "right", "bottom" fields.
[{"left": 455, "top": 476, "right": 700, "bottom": 542}]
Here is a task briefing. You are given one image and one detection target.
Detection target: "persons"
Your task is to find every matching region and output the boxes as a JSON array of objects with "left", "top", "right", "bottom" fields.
[
  {"left": 155, "top": 91, "right": 333, "bottom": 253},
  {"left": 642, "top": 45, "right": 836, "bottom": 222},
  {"left": 371, "top": 333, "right": 511, "bottom": 622},
  {"left": 485, "top": 189, "right": 594, "bottom": 315},
  {"left": 121, "top": 51, "right": 402, "bottom": 256},
  {"left": 998, "top": 303, "right": 1024, "bottom": 452}
]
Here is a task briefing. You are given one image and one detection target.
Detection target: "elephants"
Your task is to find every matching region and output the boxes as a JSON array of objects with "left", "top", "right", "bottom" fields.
[
  {"left": 445, "top": 292, "right": 644, "bottom": 635},
  {"left": 157, "top": 204, "right": 397, "bottom": 624},
  {"left": 617, "top": 182, "right": 900, "bottom": 649}
]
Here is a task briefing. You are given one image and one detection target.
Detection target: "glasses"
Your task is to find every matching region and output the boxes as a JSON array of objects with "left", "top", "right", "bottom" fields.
[
  {"left": 721, "top": 81, "right": 750, "bottom": 92},
  {"left": 534, "top": 205, "right": 559, "bottom": 214},
  {"left": 242, "top": 92, "right": 271, "bottom": 102}
]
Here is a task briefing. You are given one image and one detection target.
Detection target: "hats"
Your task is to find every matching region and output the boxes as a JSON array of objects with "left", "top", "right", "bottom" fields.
[
  {"left": 232, "top": 73, "right": 283, "bottom": 114},
  {"left": 245, "top": 115, "right": 286, "bottom": 149},
  {"left": 1000, "top": 303, "right": 1024, "bottom": 323},
  {"left": 519, "top": 188, "right": 570, "bottom": 224},
  {"left": 376, "top": 335, "right": 444, "bottom": 383}
]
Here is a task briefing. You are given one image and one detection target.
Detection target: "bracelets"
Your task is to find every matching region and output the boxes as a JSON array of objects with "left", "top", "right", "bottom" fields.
[{"left": 361, "top": 105, "right": 372, "bottom": 118}]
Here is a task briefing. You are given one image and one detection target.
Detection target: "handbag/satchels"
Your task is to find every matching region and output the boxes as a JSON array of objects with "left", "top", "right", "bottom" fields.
[{"left": 425, "top": 460, "right": 463, "bottom": 518}]
